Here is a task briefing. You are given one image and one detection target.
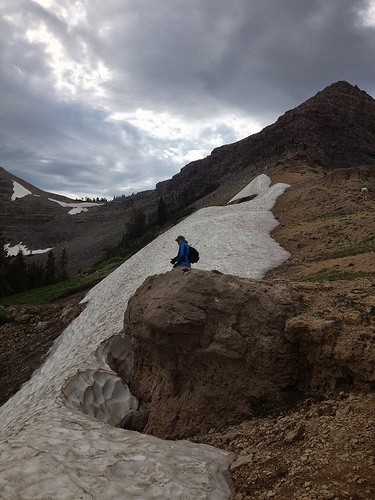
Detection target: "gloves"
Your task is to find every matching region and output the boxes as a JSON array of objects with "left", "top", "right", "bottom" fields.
[
  {"left": 173, "top": 264, "right": 177, "bottom": 268},
  {"left": 169, "top": 258, "right": 175, "bottom": 264}
]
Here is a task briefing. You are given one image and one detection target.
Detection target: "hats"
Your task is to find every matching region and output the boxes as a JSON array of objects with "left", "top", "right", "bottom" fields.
[{"left": 175, "top": 236, "right": 185, "bottom": 241}]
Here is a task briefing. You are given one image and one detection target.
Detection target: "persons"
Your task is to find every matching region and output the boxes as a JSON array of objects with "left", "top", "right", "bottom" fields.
[{"left": 169, "top": 236, "right": 192, "bottom": 268}]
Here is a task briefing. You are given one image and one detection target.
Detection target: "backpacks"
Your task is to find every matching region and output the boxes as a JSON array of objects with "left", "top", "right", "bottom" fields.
[{"left": 187, "top": 244, "right": 199, "bottom": 263}]
